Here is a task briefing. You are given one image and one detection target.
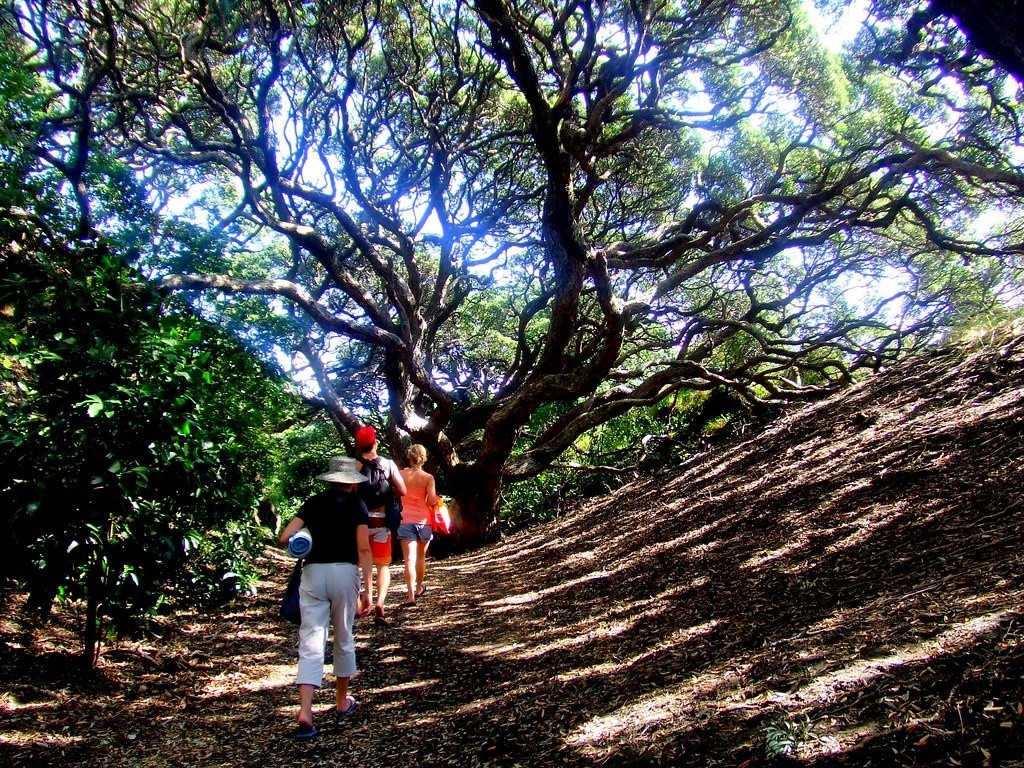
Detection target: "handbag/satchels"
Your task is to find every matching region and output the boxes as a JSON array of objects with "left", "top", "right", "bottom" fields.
[
  {"left": 432, "top": 496, "right": 450, "bottom": 534},
  {"left": 279, "top": 558, "right": 303, "bottom": 625}
]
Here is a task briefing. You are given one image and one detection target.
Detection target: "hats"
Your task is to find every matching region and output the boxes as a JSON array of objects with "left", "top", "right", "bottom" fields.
[
  {"left": 315, "top": 456, "right": 369, "bottom": 484},
  {"left": 356, "top": 426, "right": 376, "bottom": 447}
]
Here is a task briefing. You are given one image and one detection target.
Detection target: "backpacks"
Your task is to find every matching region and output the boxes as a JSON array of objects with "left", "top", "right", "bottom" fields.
[{"left": 351, "top": 456, "right": 395, "bottom": 509}]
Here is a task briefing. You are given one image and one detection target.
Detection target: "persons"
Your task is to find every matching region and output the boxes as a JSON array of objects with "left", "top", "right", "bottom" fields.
[
  {"left": 354, "top": 426, "right": 407, "bottom": 626},
  {"left": 397, "top": 444, "right": 436, "bottom": 607},
  {"left": 280, "top": 456, "right": 373, "bottom": 737}
]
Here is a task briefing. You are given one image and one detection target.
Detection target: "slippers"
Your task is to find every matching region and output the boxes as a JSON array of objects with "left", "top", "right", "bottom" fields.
[
  {"left": 373, "top": 612, "right": 385, "bottom": 625},
  {"left": 415, "top": 583, "right": 426, "bottom": 597},
  {"left": 296, "top": 724, "right": 317, "bottom": 738},
  {"left": 339, "top": 696, "right": 355, "bottom": 713},
  {"left": 404, "top": 599, "right": 416, "bottom": 607},
  {"left": 353, "top": 613, "right": 362, "bottom": 619}
]
